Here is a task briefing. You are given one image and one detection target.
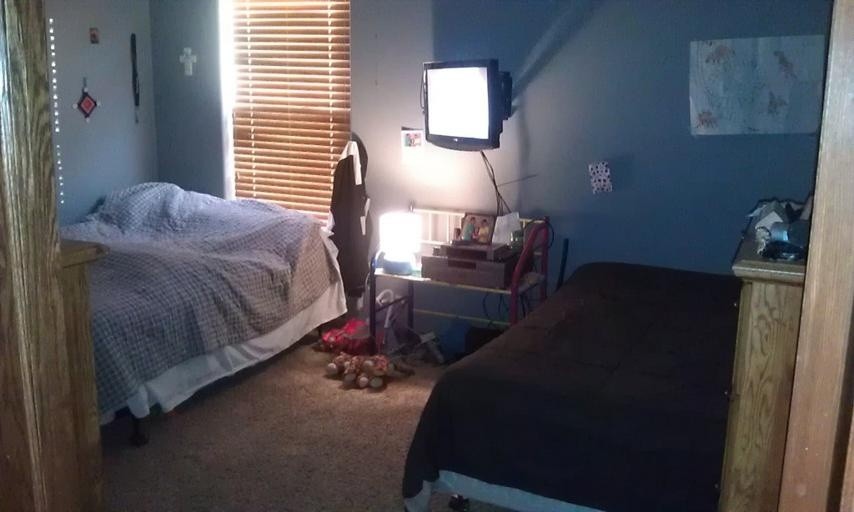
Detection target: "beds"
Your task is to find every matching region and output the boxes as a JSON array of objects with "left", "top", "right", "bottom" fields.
[
  {"left": 60, "top": 182, "right": 348, "bottom": 446},
  {"left": 403, "top": 262, "right": 742, "bottom": 512}
]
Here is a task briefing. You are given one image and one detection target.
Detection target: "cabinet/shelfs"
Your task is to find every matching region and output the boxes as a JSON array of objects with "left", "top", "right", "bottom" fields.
[{"left": 718, "top": 278, "right": 804, "bottom": 512}]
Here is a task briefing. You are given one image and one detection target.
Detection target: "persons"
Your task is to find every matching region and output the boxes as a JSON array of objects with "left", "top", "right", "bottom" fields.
[
  {"left": 460, "top": 217, "right": 493, "bottom": 246},
  {"left": 406, "top": 134, "right": 421, "bottom": 146}
]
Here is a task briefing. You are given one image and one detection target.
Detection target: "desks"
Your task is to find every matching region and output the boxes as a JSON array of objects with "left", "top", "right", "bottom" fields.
[{"left": 370, "top": 204, "right": 549, "bottom": 357}]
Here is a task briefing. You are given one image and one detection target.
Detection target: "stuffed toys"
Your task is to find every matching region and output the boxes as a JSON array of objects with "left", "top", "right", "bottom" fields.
[{"left": 328, "top": 351, "right": 416, "bottom": 390}]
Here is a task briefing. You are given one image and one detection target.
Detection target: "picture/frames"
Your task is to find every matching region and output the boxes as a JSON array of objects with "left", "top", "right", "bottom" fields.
[{"left": 456, "top": 213, "right": 497, "bottom": 245}]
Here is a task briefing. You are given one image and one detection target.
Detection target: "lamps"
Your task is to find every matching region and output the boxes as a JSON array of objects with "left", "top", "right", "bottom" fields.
[{"left": 378, "top": 211, "right": 422, "bottom": 275}]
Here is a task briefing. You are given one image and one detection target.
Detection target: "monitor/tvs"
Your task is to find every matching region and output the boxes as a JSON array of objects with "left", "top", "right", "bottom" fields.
[{"left": 419, "top": 57, "right": 513, "bottom": 156}]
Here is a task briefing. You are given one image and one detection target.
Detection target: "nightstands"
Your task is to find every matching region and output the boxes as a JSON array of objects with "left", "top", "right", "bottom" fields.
[{"left": 732, "top": 218, "right": 805, "bottom": 282}]
